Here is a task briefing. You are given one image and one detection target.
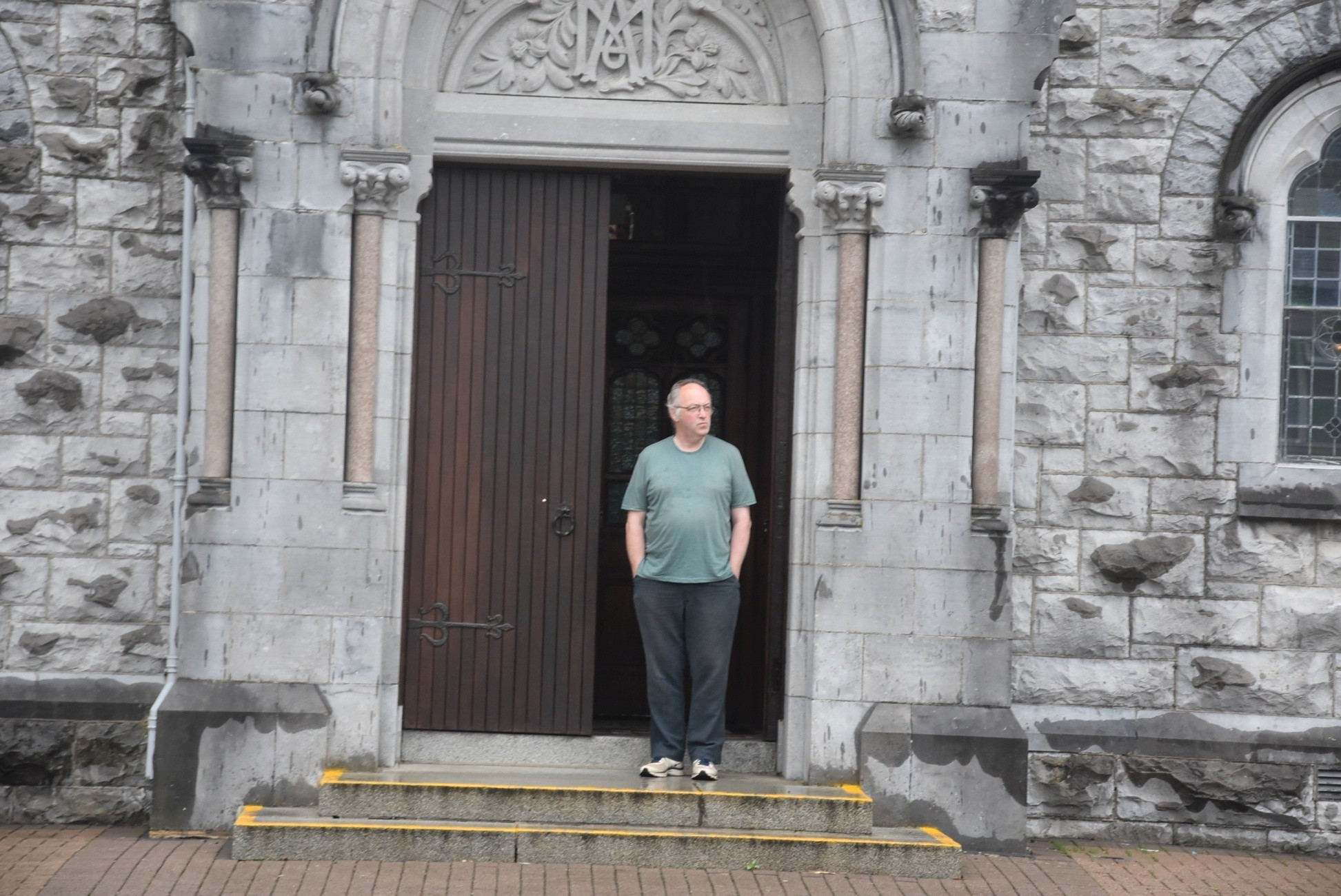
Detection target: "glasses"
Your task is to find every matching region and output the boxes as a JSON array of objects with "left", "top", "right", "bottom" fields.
[{"left": 674, "top": 402, "right": 714, "bottom": 413}]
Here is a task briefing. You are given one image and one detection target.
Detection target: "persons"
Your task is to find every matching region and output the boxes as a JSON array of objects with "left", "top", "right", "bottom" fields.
[{"left": 622, "top": 378, "right": 756, "bottom": 781}]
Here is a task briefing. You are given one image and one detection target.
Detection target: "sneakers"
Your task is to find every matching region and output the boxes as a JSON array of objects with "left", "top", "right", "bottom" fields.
[
  {"left": 639, "top": 756, "right": 685, "bottom": 778},
  {"left": 691, "top": 759, "right": 718, "bottom": 781}
]
